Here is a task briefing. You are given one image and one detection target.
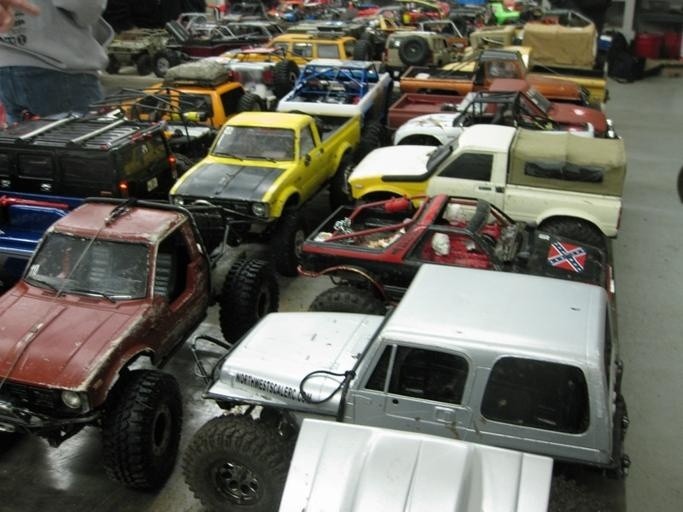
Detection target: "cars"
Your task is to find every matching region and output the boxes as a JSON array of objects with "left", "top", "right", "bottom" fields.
[{"left": 0, "top": 189, "right": 88, "bottom": 295}]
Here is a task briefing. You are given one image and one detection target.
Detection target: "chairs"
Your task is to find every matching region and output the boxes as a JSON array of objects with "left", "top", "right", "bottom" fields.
[
  {"left": 84, "top": 244, "right": 114, "bottom": 282},
  {"left": 154, "top": 250, "right": 174, "bottom": 300}
]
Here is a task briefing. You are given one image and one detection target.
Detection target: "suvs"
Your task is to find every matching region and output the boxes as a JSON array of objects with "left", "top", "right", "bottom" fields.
[
  {"left": 0, "top": 198, "right": 280, "bottom": 498},
  {"left": 189, "top": 262, "right": 630, "bottom": 485},
  {"left": 278, "top": 418, "right": 557, "bottom": 511},
  {"left": 0, "top": 0, "right": 622, "bottom": 164},
  {"left": 0, "top": 111, "right": 178, "bottom": 203}
]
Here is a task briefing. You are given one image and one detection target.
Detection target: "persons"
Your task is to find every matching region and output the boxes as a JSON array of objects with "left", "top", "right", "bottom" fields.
[{"left": 0, "top": 0, "right": 115, "bottom": 129}]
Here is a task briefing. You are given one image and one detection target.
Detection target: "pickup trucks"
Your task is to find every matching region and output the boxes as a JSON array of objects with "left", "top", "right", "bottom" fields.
[
  {"left": 345, "top": 123, "right": 628, "bottom": 253},
  {"left": 295, "top": 192, "right": 615, "bottom": 318},
  {"left": 168, "top": 108, "right": 363, "bottom": 252}
]
